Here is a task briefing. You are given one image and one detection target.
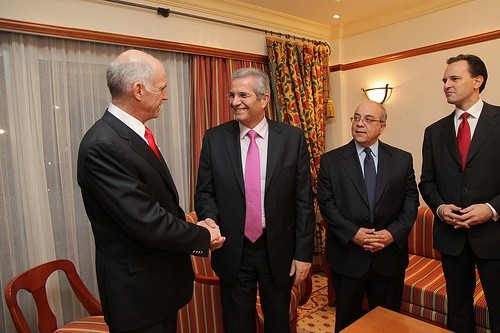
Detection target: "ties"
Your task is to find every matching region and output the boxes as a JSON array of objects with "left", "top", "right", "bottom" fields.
[
  {"left": 456, "top": 112, "right": 471, "bottom": 172},
  {"left": 363, "top": 147, "right": 377, "bottom": 226},
  {"left": 245, "top": 130, "right": 263, "bottom": 244},
  {"left": 145, "top": 126, "right": 160, "bottom": 160}
]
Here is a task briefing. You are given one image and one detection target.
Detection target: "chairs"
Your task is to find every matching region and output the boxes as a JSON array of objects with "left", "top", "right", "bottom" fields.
[{"left": 5, "top": 210, "right": 313, "bottom": 333}]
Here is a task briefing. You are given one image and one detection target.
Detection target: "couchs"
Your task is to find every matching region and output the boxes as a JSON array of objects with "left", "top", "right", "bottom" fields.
[{"left": 320, "top": 206, "right": 490, "bottom": 333}]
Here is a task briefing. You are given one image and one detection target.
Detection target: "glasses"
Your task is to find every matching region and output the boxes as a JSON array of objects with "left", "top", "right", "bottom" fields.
[
  {"left": 226, "top": 92, "right": 255, "bottom": 99},
  {"left": 350, "top": 116, "right": 385, "bottom": 123}
]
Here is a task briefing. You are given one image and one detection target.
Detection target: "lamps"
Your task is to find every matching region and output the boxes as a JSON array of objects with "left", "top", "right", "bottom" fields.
[{"left": 361, "top": 83, "right": 393, "bottom": 105}]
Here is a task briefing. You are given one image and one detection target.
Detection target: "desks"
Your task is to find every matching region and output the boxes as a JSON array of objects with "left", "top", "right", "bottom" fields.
[{"left": 339, "top": 306, "right": 455, "bottom": 333}]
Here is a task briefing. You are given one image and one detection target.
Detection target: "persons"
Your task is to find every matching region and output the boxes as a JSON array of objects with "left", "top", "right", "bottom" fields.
[
  {"left": 76, "top": 48, "right": 221, "bottom": 333},
  {"left": 317, "top": 101, "right": 419, "bottom": 333},
  {"left": 419, "top": 54, "right": 500, "bottom": 333},
  {"left": 194, "top": 67, "right": 317, "bottom": 333}
]
